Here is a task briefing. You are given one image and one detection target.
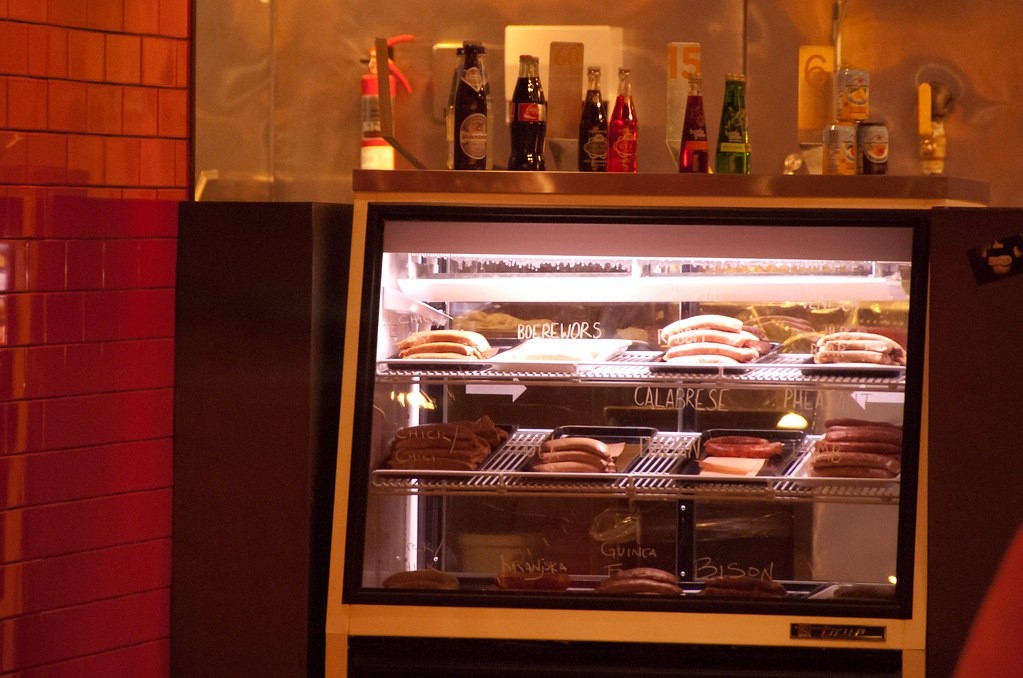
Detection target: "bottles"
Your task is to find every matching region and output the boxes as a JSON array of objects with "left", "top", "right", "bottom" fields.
[
  {"left": 445, "top": 40, "right": 493, "bottom": 170},
  {"left": 508, "top": 54, "right": 548, "bottom": 171},
  {"left": 579, "top": 66, "right": 609, "bottom": 172},
  {"left": 606, "top": 68, "right": 639, "bottom": 173},
  {"left": 679, "top": 72, "right": 709, "bottom": 173},
  {"left": 716, "top": 72, "right": 751, "bottom": 173}
]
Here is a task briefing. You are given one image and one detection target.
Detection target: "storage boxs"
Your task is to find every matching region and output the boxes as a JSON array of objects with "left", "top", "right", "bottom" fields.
[{"left": 306, "top": 171, "right": 994, "bottom": 678}]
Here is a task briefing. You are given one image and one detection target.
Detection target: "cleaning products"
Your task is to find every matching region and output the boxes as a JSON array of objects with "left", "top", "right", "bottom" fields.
[{"left": 357, "top": 32, "right": 414, "bottom": 171}]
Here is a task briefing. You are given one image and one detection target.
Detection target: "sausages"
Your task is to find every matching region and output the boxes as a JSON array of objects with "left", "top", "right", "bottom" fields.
[{"left": 382, "top": 313, "right": 903, "bottom": 599}]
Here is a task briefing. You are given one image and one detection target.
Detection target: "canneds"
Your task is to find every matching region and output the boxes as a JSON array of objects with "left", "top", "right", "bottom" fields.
[
  {"left": 821, "top": 123, "right": 856, "bottom": 175},
  {"left": 858, "top": 120, "right": 889, "bottom": 175},
  {"left": 835, "top": 65, "right": 869, "bottom": 121}
]
